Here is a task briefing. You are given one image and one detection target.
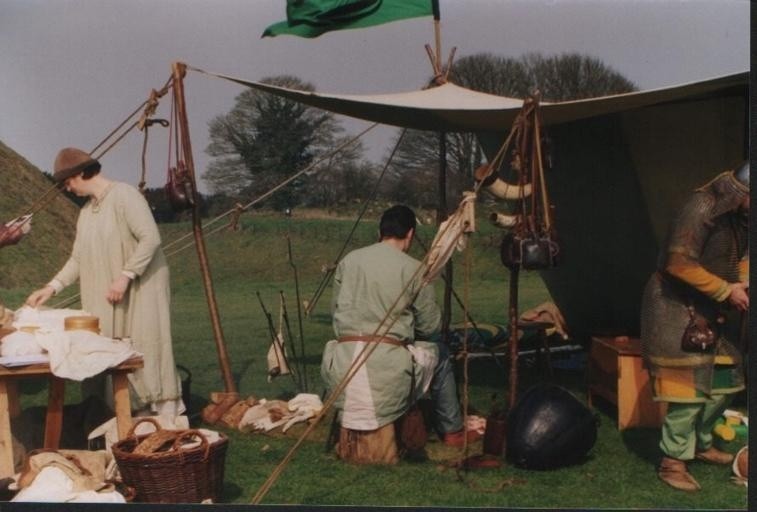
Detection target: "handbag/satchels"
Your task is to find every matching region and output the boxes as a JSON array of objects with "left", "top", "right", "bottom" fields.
[{"left": 680, "top": 316, "right": 724, "bottom": 352}]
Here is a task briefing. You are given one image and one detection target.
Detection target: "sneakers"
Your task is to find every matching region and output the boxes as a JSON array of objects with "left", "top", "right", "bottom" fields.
[
  {"left": 658, "top": 456, "right": 702, "bottom": 492},
  {"left": 444, "top": 427, "right": 480, "bottom": 449},
  {"left": 695, "top": 446, "right": 734, "bottom": 465}
]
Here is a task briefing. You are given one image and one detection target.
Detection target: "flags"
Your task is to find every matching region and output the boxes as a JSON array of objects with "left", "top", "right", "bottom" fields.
[{"left": 261, "top": 1, "right": 439, "bottom": 41}]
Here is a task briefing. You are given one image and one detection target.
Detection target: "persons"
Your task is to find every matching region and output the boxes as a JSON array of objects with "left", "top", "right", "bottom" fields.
[
  {"left": 637, "top": 159, "right": 750, "bottom": 493},
  {"left": 27, "top": 147, "right": 189, "bottom": 421},
  {"left": 317, "top": 204, "right": 486, "bottom": 448}
]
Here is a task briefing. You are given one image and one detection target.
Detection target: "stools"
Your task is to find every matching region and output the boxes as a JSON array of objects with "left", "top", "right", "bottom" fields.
[{"left": 332, "top": 417, "right": 402, "bottom": 467}]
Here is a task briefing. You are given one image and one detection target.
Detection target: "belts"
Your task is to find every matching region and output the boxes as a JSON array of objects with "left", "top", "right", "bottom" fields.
[{"left": 337, "top": 334, "right": 400, "bottom": 346}]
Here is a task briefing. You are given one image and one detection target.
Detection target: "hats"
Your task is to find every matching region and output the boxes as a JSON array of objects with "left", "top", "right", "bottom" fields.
[{"left": 53, "top": 147, "right": 95, "bottom": 185}]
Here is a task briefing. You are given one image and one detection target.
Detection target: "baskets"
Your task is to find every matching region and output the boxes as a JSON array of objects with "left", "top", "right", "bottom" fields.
[{"left": 111, "top": 420, "right": 230, "bottom": 504}]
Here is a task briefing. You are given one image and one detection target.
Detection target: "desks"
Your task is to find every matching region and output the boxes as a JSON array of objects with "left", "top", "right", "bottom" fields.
[
  {"left": 588, "top": 332, "right": 671, "bottom": 430},
  {"left": 0, "top": 345, "right": 145, "bottom": 479}
]
